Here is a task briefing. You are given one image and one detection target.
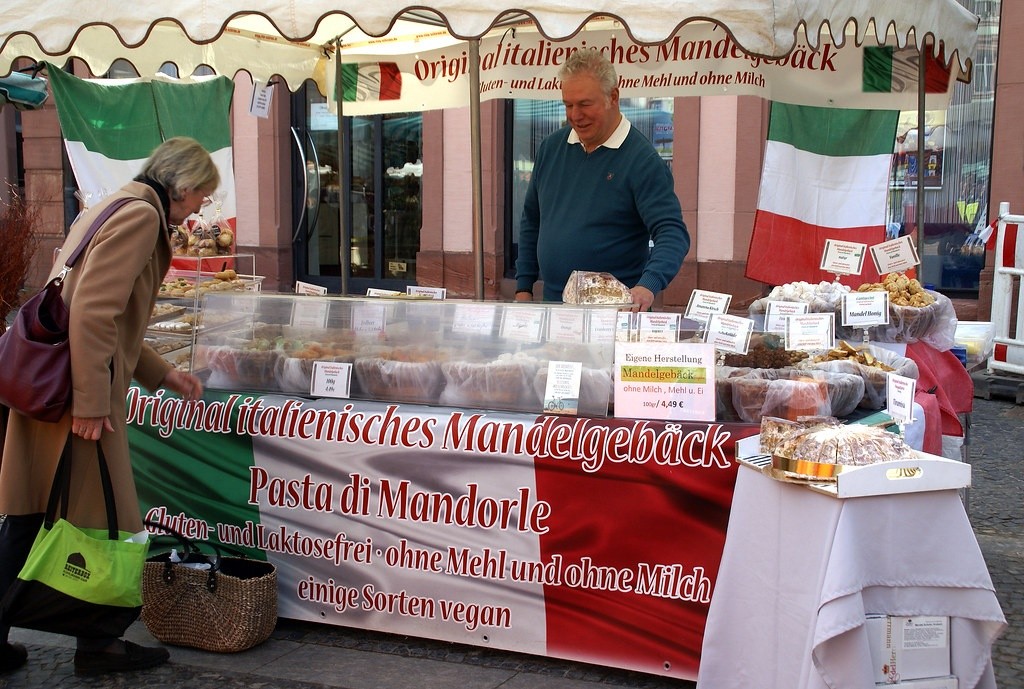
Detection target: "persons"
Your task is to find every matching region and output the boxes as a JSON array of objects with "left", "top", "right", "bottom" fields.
[
  {"left": 515, "top": 48, "right": 690, "bottom": 313},
  {"left": 0, "top": 136, "right": 223, "bottom": 675}
]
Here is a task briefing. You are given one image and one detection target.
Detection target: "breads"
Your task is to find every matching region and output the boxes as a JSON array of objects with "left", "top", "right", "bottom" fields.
[
  {"left": 144, "top": 269, "right": 245, "bottom": 373},
  {"left": 856, "top": 271, "right": 934, "bottom": 310},
  {"left": 168, "top": 224, "right": 234, "bottom": 257}
]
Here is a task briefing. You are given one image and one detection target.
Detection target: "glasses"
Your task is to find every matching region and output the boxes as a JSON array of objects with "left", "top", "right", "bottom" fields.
[{"left": 198, "top": 188, "right": 212, "bottom": 208}]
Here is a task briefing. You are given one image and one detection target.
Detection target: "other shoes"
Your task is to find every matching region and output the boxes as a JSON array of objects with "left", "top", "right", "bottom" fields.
[
  {"left": 0, "top": 642, "right": 28, "bottom": 670},
  {"left": 74, "top": 640, "right": 169, "bottom": 677}
]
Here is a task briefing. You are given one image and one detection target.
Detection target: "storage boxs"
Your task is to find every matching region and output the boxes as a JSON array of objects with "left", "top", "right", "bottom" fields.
[{"left": 202, "top": 288, "right": 948, "bottom": 425}]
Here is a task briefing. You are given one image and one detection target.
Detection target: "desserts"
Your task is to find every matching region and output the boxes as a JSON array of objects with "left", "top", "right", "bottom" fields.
[{"left": 769, "top": 279, "right": 850, "bottom": 302}]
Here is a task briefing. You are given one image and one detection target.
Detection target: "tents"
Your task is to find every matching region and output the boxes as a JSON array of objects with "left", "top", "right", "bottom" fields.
[{"left": 1, "top": 0, "right": 985, "bottom": 303}]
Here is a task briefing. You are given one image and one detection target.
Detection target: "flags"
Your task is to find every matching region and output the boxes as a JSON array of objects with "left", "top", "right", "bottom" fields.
[
  {"left": 745, "top": 100, "right": 900, "bottom": 290},
  {"left": 41, "top": 60, "right": 238, "bottom": 272}
]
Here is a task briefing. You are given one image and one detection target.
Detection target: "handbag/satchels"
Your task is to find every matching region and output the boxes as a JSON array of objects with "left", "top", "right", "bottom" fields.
[
  {"left": 0, "top": 432, "right": 150, "bottom": 638},
  {"left": 0, "top": 276, "right": 72, "bottom": 423},
  {"left": 140, "top": 520, "right": 280, "bottom": 652}
]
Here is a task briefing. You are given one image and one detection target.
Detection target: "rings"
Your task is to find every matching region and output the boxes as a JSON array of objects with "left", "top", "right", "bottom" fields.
[{"left": 80, "top": 429, "right": 87, "bottom": 432}]
